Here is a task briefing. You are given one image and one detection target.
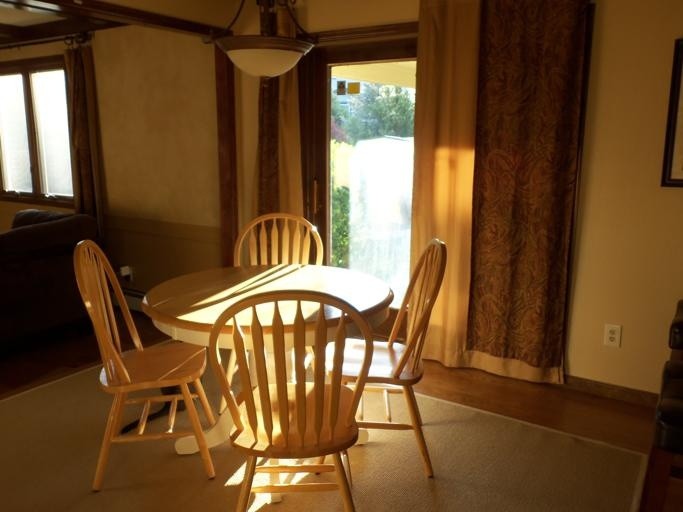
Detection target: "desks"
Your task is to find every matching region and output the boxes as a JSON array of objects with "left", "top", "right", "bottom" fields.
[{"left": 139, "top": 262, "right": 394, "bottom": 504}]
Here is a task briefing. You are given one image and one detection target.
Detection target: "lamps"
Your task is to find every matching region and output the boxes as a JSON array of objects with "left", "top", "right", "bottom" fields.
[{"left": 200, "top": 0, "right": 317, "bottom": 79}]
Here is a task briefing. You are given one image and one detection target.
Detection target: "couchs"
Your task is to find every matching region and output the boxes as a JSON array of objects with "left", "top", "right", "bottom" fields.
[{"left": 1, "top": 208, "right": 96, "bottom": 365}]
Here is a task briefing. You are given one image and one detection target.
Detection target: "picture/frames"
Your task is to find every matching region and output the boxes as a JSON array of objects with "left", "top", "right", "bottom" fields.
[{"left": 661, "top": 37, "right": 683, "bottom": 188}]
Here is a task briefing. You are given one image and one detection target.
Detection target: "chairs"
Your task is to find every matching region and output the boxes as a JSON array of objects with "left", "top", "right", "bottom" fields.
[
  {"left": 313, "top": 236, "right": 448, "bottom": 479},
  {"left": 216, "top": 214, "right": 324, "bottom": 416},
  {"left": 208, "top": 288, "right": 374, "bottom": 511},
  {"left": 651, "top": 298, "right": 683, "bottom": 453},
  {"left": 72, "top": 238, "right": 227, "bottom": 494}
]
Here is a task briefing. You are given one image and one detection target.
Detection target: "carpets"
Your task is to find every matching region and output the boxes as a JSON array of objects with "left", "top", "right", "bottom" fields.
[{"left": 2, "top": 337, "right": 650, "bottom": 510}]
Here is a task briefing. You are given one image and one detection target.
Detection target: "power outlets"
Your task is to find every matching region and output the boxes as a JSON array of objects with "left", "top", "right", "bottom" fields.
[{"left": 603, "top": 323, "right": 622, "bottom": 347}]
